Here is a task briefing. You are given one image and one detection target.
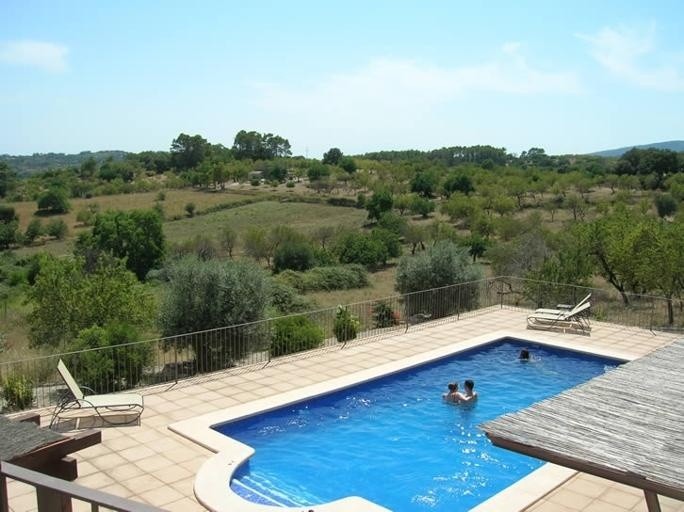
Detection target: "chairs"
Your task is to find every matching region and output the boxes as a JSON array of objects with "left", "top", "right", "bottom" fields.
[
  {"left": 535, "top": 292, "right": 594, "bottom": 330},
  {"left": 45, "top": 356, "right": 145, "bottom": 434},
  {"left": 526, "top": 301, "right": 593, "bottom": 336}
]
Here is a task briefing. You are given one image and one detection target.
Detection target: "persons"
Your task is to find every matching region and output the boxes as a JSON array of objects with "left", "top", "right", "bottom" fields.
[
  {"left": 462, "top": 379, "right": 477, "bottom": 405},
  {"left": 442, "top": 382, "right": 476, "bottom": 404},
  {"left": 520, "top": 349, "right": 530, "bottom": 361}
]
